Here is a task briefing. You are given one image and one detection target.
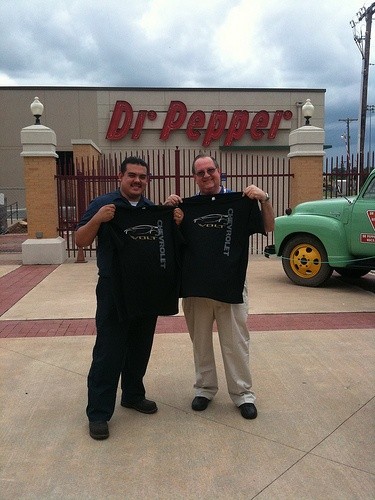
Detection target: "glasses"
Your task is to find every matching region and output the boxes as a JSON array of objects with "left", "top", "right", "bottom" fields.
[{"left": 197, "top": 167, "right": 217, "bottom": 177}]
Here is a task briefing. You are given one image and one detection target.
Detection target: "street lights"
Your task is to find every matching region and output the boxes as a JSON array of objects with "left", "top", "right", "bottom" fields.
[{"left": 366, "top": 105, "right": 375, "bottom": 168}]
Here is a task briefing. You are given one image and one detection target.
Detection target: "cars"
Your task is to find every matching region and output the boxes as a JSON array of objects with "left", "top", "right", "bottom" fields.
[{"left": 265, "top": 169, "right": 375, "bottom": 288}]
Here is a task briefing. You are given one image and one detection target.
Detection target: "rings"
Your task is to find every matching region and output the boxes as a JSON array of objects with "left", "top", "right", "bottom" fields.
[{"left": 178, "top": 215, "right": 182, "bottom": 217}]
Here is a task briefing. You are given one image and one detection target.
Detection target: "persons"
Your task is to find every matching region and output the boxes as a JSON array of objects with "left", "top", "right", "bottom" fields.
[
  {"left": 73, "top": 157, "right": 183, "bottom": 440},
  {"left": 166, "top": 154, "right": 274, "bottom": 419}
]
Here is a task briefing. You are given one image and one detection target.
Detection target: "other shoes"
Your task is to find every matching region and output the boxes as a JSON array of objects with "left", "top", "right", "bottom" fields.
[
  {"left": 191, "top": 396, "right": 210, "bottom": 411},
  {"left": 240, "top": 403, "right": 258, "bottom": 419},
  {"left": 121, "top": 398, "right": 158, "bottom": 414},
  {"left": 89, "top": 419, "right": 109, "bottom": 441}
]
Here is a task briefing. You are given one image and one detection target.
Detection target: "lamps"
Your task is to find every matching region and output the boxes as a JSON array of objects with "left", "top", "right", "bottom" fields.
[
  {"left": 30, "top": 96, "right": 44, "bottom": 125},
  {"left": 302, "top": 98, "right": 314, "bottom": 125}
]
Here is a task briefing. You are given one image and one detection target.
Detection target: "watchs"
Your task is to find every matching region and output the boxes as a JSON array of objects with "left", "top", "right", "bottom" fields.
[{"left": 260, "top": 193, "right": 271, "bottom": 204}]
